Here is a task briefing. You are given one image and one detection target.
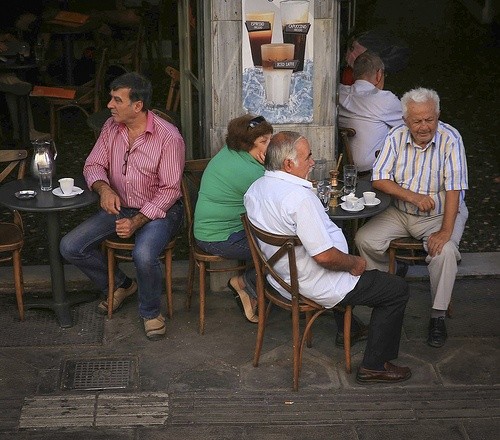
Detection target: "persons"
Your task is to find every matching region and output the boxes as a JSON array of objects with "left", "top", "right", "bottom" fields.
[
  {"left": 61, "top": 72, "right": 185, "bottom": 341},
  {"left": 338, "top": 37, "right": 469, "bottom": 348},
  {"left": 243, "top": 130, "right": 413, "bottom": 383},
  {"left": 192, "top": 116, "right": 275, "bottom": 324}
]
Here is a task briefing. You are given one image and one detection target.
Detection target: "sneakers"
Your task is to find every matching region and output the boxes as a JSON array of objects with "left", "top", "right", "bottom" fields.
[
  {"left": 95, "top": 279, "right": 138, "bottom": 314},
  {"left": 144, "top": 313, "right": 167, "bottom": 340}
]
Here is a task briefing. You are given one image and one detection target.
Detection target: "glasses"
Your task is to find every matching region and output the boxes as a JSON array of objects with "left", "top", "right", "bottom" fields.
[
  {"left": 121, "top": 151, "right": 129, "bottom": 176},
  {"left": 247, "top": 116, "right": 266, "bottom": 132},
  {"left": 377, "top": 68, "right": 388, "bottom": 77}
]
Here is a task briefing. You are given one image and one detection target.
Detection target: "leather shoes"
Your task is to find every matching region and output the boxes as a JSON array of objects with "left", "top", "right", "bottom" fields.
[
  {"left": 356, "top": 361, "right": 411, "bottom": 384},
  {"left": 335, "top": 323, "right": 369, "bottom": 348},
  {"left": 394, "top": 260, "right": 409, "bottom": 278},
  {"left": 428, "top": 317, "right": 447, "bottom": 346}
]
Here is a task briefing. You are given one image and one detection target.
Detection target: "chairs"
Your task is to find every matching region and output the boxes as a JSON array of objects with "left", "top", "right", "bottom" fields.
[{"left": 0, "top": 7, "right": 430, "bottom": 393}]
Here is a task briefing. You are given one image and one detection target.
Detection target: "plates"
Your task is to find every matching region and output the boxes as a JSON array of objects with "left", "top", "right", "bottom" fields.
[
  {"left": 15, "top": 190, "right": 37, "bottom": 199},
  {"left": 359, "top": 197, "right": 381, "bottom": 207},
  {"left": 341, "top": 202, "right": 365, "bottom": 213},
  {"left": 52, "top": 186, "right": 84, "bottom": 199}
]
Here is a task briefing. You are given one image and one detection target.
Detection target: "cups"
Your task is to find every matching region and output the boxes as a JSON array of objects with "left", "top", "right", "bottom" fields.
[
  {"left": 280, "top": 0, "right": 311, "bottom": 74},
  {"left": 59, "top": 178, "right": 74, "bottom": 194},
  {"left": 316, "top": 181, "right": 331, "bottom": 212},
  {"left": 34, "top": 42, "right": 48, "bottom": 65},
  {"left": 261, "top": 43, "right": 295, "bottom": 108},
  {"left": 38, "top": 161, "right": 53, "bottom": 191},
  {"left": 345, "top": 197, "right": 358, "bottom": 209},
  {"left": 362, "top": 191, "right": 376, "bottom": 204},
  {"left": 245, "top": 10, "right": 275, "bottom": 68},
  {"left": 343, "top": 165, "right": 357, "bottom": 194}
]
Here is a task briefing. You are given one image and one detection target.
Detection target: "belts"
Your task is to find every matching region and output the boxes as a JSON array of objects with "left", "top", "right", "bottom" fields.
[{"left": 357, "top": 169, "right": 373, "bottom": 177}]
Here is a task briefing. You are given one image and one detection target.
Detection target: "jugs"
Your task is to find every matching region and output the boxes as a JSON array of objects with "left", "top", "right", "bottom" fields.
[{"left": 32, "top": 136, "right": 58, "bottom": 181}]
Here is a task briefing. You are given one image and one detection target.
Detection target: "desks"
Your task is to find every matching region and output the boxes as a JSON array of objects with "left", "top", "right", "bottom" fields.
[
  {"left": 102, "top": 8, "right": 142, "bottom": 48},
  {"left": 86, "top": 105, "right": 181, "bottom": 141},
  {"left": 310, "top": 177, "right": 392, "bottom": 255},
  {"left": 27, "top": 13, "right": 102, "bottom": 87},
  {"left": 0, "top": 176, "right": 100, "bottom": 328},
  {"left": 0, "top": 39, "right": 63, "bottom": 155}
]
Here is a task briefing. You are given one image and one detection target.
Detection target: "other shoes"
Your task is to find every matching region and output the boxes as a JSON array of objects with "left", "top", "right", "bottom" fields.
[{"left": 228, "top": 277, "right": 258, "bottom": 323}]
